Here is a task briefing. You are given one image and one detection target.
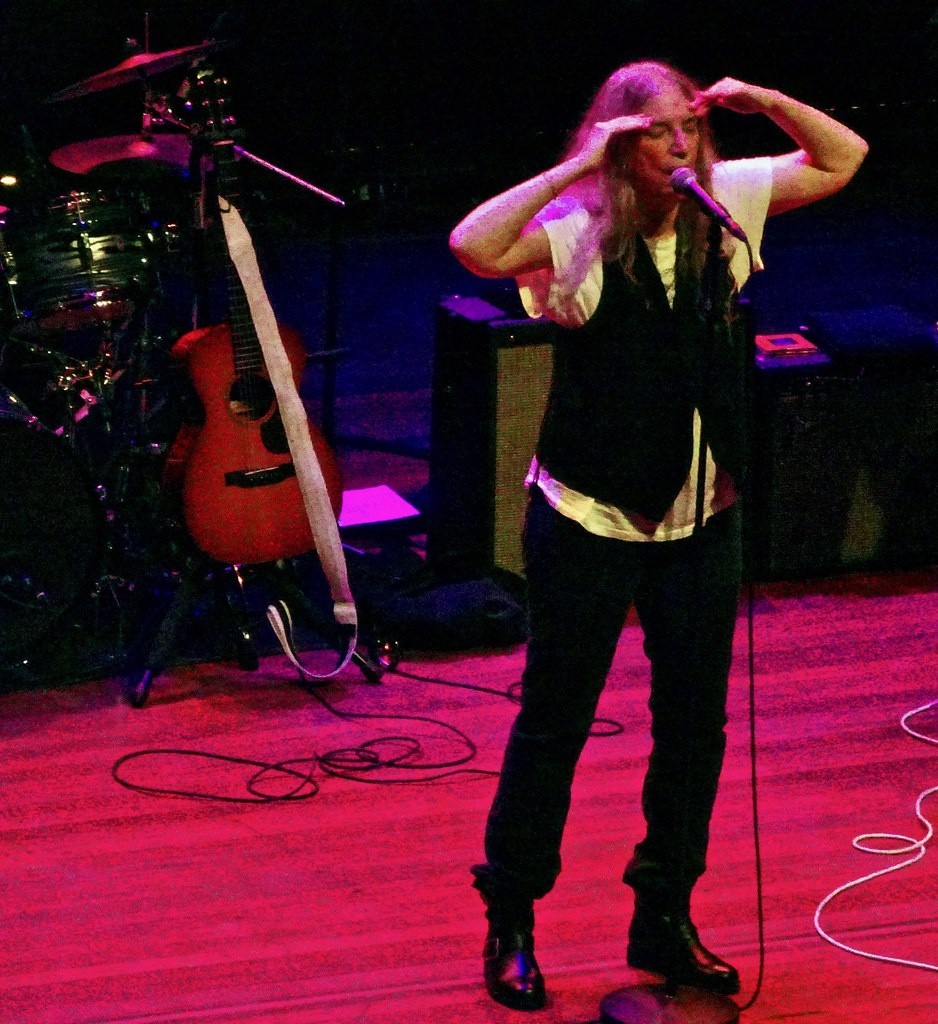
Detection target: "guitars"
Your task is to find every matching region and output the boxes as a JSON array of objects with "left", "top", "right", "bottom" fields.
[{"left": 173, "top": 56, "right": 342, "bottom": 565}]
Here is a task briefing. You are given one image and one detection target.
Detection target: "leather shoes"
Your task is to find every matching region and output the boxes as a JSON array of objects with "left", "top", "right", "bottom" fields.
[
  {"left": 481, "top": 926, "right": 546, "bottom": 1011},
  {"left": 626, "top": 914, "right": 742, "bottom": 994}
]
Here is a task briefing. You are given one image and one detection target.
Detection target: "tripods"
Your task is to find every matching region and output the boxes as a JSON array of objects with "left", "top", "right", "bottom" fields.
[{"left": 128, "top": 130, "right": 384, "bottom": 708}]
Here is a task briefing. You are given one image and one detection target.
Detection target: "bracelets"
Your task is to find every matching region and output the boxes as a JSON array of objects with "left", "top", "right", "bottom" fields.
[{"left": 543, "top": 169, "right": 559, "bottom": 201}]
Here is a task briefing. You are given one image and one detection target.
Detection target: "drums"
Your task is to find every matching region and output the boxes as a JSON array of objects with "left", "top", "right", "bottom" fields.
[{"left": 0, "top": 183, "right": 162, "bottom": 332}]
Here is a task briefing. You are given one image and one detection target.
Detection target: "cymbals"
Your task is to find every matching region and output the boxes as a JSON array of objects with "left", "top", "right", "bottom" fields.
[
  {"left": 47, "top": 36, "right": 221, "bottom": 101},
  {"left": 49, "top": 132, "right": 242, "bottom": 175}
]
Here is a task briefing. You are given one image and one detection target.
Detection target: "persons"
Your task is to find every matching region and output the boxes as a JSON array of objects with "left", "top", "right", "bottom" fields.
[{"left": 444, "top": 60, "right": 868, "bottom": 1011}]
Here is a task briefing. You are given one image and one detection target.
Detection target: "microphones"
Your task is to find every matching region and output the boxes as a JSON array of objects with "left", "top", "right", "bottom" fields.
[{"left": 671, "top": 166, "right": 748, "bottom": 241}]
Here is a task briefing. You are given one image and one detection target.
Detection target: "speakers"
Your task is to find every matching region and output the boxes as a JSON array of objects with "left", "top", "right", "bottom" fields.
[
  {"left": 758, "top": 302, "right": 938, "bottom": 582},
  {"left": 431, "top": 297, "right": 554, "bottom": 604}
]
